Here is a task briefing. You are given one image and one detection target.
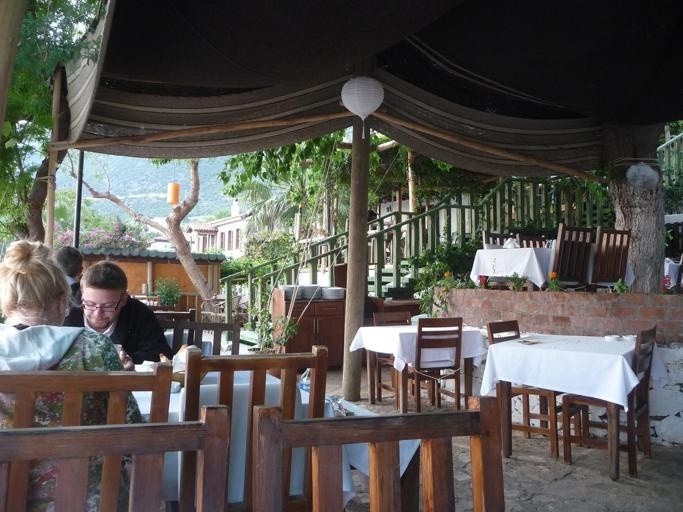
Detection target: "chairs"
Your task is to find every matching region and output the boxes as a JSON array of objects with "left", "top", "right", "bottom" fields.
[
  {"left": 469, "top": 221, "right": 634, "bottom": 292},
  {"left": 0, "top": 305, "right": 683, "bottom": 512}
]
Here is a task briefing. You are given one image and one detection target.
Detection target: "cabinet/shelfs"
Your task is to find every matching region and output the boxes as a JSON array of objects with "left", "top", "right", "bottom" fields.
[{"left": 270, "top": 285, "right": 344, "bottom": 370}]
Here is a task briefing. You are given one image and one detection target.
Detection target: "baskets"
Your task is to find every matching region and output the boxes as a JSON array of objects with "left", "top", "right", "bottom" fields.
[{"left": 153, "top": 359, "right": 208, "bottom": 387}]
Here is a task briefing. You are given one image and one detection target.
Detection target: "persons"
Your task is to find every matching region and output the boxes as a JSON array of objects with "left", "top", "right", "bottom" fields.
[
  {"left": 61, "top": 261, "right": 171, "bottom": 362},
  {"left": 53, "top": 246, "right": 85, "bottom": 327},
  {"left": 0, "top": 241, "right": 144, "bottom": 511}
]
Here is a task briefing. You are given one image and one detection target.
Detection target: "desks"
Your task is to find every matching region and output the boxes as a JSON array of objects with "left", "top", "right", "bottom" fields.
[
  {"left": 348, "top": 322, "right": 489, "bottom": 416},
  {"left": 478, "top": 332, "right": 641, "bottom": 482},
  {"left": 129, "top": 364, "right": 356, "bottom": 506}
]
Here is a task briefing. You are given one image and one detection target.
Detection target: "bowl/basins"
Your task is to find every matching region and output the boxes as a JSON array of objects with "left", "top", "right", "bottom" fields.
[
  {"left": 602, "top": 333, "right": 620, "bottom": 343},
  {"left": 623, "top": 333, "right": 638, "bottom": 344}
]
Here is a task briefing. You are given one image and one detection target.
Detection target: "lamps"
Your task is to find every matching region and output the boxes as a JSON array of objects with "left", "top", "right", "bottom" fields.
[{"left": 166, "top": 158, "right": 182, "bottom": 207}]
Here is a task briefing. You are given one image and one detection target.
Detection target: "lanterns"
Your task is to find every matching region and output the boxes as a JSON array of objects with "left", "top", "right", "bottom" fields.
[{"left": 165, "top": 182, "right": 180, "bottom": 205}]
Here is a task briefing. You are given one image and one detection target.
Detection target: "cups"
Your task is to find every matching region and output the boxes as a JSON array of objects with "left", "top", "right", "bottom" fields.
[{"left": 112, "top": 343, "right": 121, "bottom": 358}]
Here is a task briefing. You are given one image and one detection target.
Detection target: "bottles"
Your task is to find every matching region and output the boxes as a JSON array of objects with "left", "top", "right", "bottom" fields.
[{"left": 298, "top": 367, "right": 309, "bottom": 397}]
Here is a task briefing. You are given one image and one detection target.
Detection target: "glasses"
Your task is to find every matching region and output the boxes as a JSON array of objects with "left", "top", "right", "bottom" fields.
[{"left": 81, "top": 293, "right": 124, "bottom": 312}]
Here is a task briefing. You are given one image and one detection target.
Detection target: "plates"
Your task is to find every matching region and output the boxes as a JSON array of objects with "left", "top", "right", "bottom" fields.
[{"left": 276, "top": 281, "right": 347, "bottom": 303}]
